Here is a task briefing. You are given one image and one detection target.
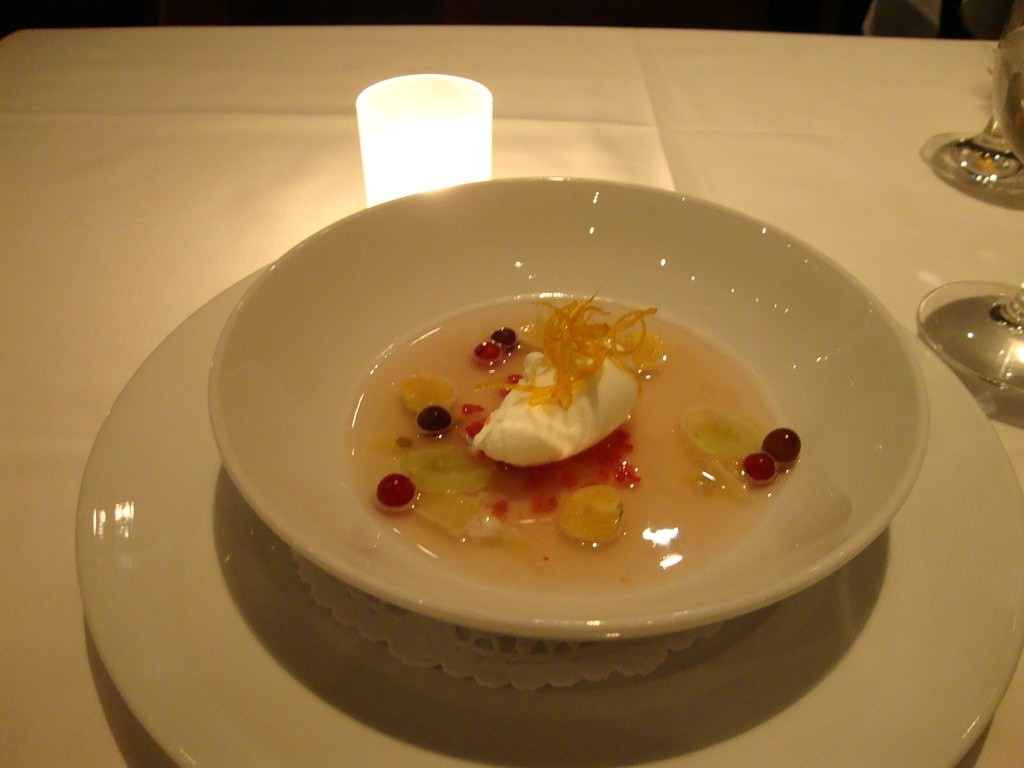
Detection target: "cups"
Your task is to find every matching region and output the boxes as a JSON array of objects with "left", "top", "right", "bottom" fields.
[{"left": 355, "top": 73, "right": 494, "bottom": 208}]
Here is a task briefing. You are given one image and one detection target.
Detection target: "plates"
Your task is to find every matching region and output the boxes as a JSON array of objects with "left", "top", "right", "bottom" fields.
[{"left": 76, "top": 267, "right": 1024, "bottom": 768}]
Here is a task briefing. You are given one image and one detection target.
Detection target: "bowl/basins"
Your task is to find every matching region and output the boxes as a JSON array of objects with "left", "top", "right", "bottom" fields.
[{"left": 211, "top": 177, "right": 929, "bottom": 634}]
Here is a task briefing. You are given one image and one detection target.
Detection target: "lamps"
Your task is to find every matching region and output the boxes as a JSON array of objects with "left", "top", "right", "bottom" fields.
[{"left": 354, "top": 73, "right": 495, "bottom": 216}]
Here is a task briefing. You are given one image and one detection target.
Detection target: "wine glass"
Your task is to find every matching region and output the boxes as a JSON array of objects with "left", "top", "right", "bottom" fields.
[
  {"left": 916, "top": 0, "right": 1024, "bottom": 395},
  {"left": 920, "top": 0, "right": 1024, "bottom": 195}
]
